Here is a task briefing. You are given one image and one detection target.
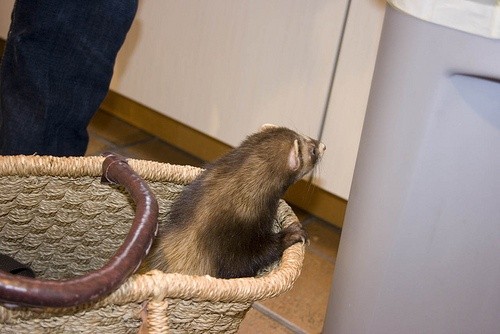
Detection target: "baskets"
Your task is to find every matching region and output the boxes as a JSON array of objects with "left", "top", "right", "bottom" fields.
[{"left": 0, "top": 154, "right": 307, "bottom": 334}]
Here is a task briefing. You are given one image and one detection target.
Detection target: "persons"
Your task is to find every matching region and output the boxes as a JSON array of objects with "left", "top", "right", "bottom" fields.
[{"left": 2, "top": 0, "right": 142, "bottom": 157}]
